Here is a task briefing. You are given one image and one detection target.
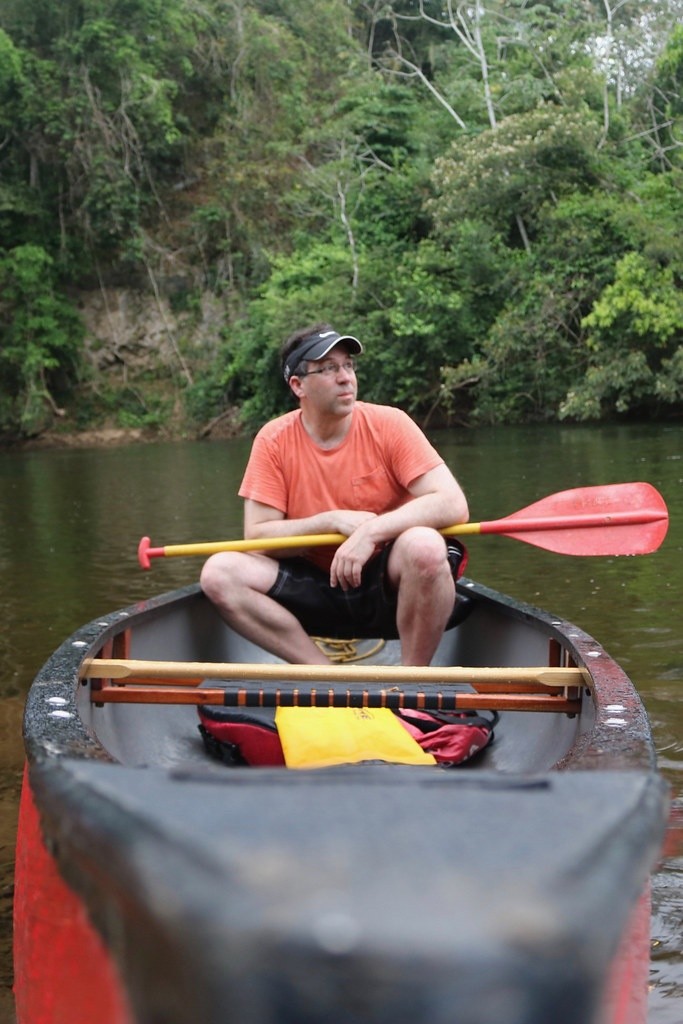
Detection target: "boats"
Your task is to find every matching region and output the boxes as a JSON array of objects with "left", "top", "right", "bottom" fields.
[{"left": 13, "top": 575, "right": 673, "bottom": 1024}]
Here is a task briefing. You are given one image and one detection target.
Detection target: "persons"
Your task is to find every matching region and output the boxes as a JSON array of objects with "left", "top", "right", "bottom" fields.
[{"left": 200, "top": 322, "right": 470, "bottom": 667}]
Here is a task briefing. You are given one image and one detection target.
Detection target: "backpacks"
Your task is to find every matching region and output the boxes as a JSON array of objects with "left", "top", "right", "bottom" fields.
[{"left": 197, "top": 703, "right": 501, "bottom": 768}]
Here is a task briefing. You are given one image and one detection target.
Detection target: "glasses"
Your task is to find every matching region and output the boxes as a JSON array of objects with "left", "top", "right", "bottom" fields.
[{"left": 297, "top": 357, "right": 358, "bottom": 378}]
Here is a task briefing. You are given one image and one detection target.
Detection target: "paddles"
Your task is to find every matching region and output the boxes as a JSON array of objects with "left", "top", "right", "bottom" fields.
[{"left": 136, "top": 479, "right": 671, "bottom": 571}]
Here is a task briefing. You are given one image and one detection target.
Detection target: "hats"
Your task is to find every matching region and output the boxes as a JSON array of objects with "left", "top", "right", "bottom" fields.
[{"left": 284, "top": 328, "right": 363, "bottom": 383}]
